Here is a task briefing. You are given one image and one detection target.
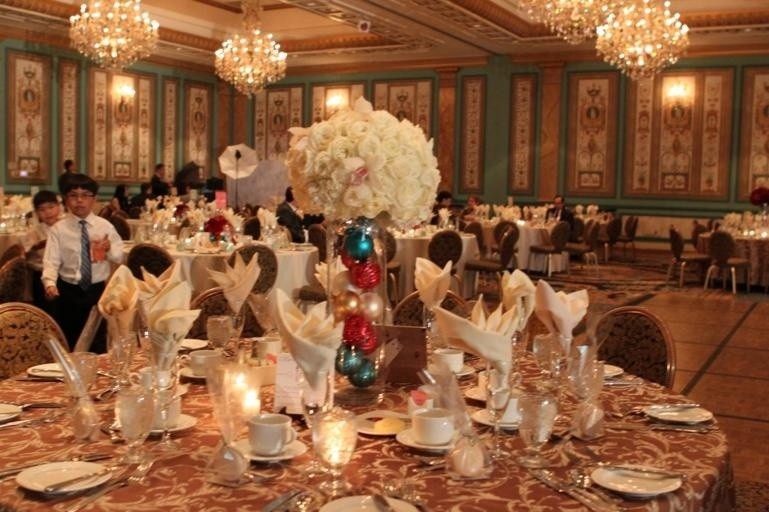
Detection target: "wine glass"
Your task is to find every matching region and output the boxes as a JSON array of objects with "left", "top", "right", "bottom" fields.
[{"left": 312, "top": 411, "right": 358, "bottom": 496}]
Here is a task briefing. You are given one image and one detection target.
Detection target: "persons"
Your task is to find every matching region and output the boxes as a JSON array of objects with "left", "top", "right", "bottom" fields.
[
  {"left": 431, "top": 191, "right": 452, "bottom": 225},
  {"left": 57, "top": 160, "right": 74, "bottom": 199},
  {"left": 41, "top": 173, "right": 123, "bottom": 355},
  {"left": 505, "top": 196, "right": 513, "bottom": 207},
  {"left": 545, "top": 195, "right": 573, "bottom": 222},
  {"left": 114, "top": 163, "right": 169, "bottom": 210},
  {"left": 276, "top": 185, "right": 325, "bottom": 242},
  {"left": 26, "top": 190, "right": 58, "bottom": 252},
  {"left": 468, "top": 196, "right": 482, "bottom": 205},
  {"left": 521, "top": 205, "right": 532, "bottom": 221}
]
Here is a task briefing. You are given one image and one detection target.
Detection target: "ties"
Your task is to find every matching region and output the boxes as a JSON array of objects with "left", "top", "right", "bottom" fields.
[
  {"left": 78, "top": 220, "right": 92, "bottom": 291},
  {"left": 555, "top": 209, "right": 559, "bottom": 218}
]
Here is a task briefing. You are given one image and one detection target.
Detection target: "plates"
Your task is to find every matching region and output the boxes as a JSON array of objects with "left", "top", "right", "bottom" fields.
[
  {"left": 357, "top": 407, "right": 408, "bottom": 438},
  {"left": 14, "top": 460, "right": 112, "bottom": 496},
  {"left": 605, "top": 365, "right": 624, "bottom": 380},
  {"left": 227, "top": 440, "right": 307, "bottom": 463},
  {"left": 315, "top": 495, "right": 419, "bottom": 511},
  {"left": 398, "top": 428, "right": 456, "bottom": 452},
  {"left": 640, "top": 405, "right": 715, "bottom": 424},
  {"left": 27, "top": 363, "right": 64, "bottom": 378},
  {"left": 0, "top": 404, "right": 23, "bottom": 425},
  {"left": 592, "top": 465, "right": 682, "bottom": 498}
]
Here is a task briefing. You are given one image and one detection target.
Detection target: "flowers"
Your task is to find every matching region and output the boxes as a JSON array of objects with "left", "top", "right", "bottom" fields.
[{"left": 284, "top": 97, "right": 444, "bottom": 235}]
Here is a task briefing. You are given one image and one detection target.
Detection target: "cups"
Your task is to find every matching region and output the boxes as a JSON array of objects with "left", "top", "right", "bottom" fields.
[
  {"left": 60, "top": 316, "right": 297, "bottom": 484},
  {"left": 411, "top": 408, "right": 455, "bottom": 446},
  {"left": 131, "top": 222, "right": 253, "bottom": 253},
  {"left": 91, "top": 236, "right": 106, "bottom": 263},
  {"left": 432, "top": 329, "right": 606, "bottom": 465}
]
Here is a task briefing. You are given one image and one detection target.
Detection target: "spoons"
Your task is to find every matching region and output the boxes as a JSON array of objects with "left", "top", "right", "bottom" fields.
[
  {"left": 412, "top": 454, "right": 447, "bottom": 467},
  {"left": 593, "top": 460, "right": 682, "bottom": 479},
  {"left": 568, "top": 469, "right": 618, "bottom": 505}
]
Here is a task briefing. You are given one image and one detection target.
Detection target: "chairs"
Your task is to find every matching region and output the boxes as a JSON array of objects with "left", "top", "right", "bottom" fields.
[
  {"left": 664, "top": 212, "right": 769, "bottom": 298},
  {"left": 1, "top": 181, "right": 641, "bottom": 318},
  {"left": 594, "top": 306, "right": 677, "bottom": 390}
]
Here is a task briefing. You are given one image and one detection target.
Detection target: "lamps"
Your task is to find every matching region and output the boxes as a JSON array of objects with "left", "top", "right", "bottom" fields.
[
  {"left": 592, "top": 0, "right": 692, "bottom": 85},
  {"left": 516, "top": 1, "right": 611, "bottom": 50},
  {"left": 212, "top": 1, "right": 290, "bottom": 102},
  {"left": 67, "top": 1, "right": 161, "bottom": 73}
]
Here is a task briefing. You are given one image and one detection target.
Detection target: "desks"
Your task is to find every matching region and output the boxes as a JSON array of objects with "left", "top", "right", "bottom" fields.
[{"left": 1, "top": 318, "right": 732, "bottom": 512}]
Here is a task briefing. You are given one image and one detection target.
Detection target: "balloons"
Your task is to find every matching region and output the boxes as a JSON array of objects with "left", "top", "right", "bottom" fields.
[{"left": 334, "top": 219, "right": 387, "bottom": 386}]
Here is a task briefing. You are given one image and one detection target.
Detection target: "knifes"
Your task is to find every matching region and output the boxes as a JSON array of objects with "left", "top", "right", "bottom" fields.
[
  {"left": 68, "top": 480, "right": 127, "bottom": 511},
  {"left": 28, "top": 401, "right": 65, "bottom": 408},
  {"left": 642, "top": 403, "right": 700, "bottom": 413},
  {"left": 43, "top": 464, "right": 127, "bottom": 493},
  {"left": 374, "top": 493, "right": 395, "bottom": 512},
  {"left": 603, "top": 422, "right": 704, "bottom": 434},
  {"left": 525, "top": 467, "right": 603, "bottom": 510},
  {"left": 262, "top": 488, "right": 302, "bottom": 511}
]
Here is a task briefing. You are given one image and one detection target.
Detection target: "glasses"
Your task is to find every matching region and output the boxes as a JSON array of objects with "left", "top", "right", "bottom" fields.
[{"left": 67, "top": 192, "right": 94, "bottom": 200}]
